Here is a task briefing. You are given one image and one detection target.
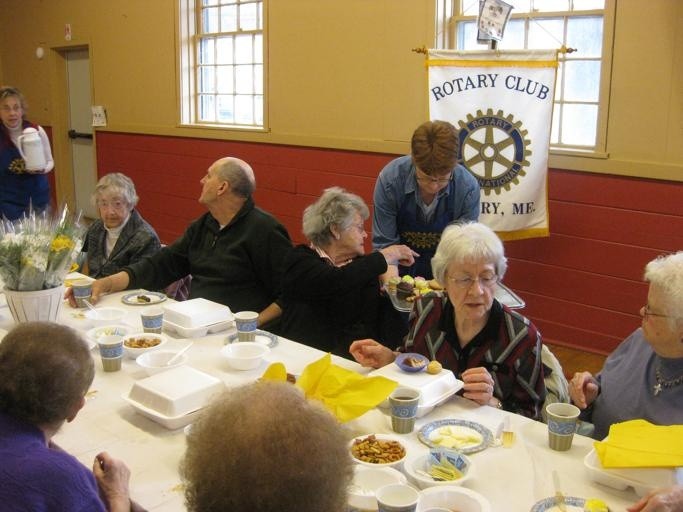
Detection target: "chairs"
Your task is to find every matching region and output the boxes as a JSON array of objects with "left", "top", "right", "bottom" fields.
[
  {"left": 160, "top": 244, "right": 192, "bottom": 301},
  {"left": 540, "top": 344, "right": 572, "bottom": 425}
]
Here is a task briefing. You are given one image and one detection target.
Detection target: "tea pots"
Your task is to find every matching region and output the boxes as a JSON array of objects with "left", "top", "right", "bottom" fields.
[{"left": 16, "top": 126, "right": 47, "bottom": 171}]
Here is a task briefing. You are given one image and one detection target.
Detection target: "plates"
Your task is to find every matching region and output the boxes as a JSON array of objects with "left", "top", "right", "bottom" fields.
[
  {"left": 122, "top": 289, "right": 167, "bottom": 305},
  {"left": 531, "top": 496, "right": 611, "bottom": 512},
  {"left": 418, "top": 418, "right": 495, "bottom": 455},
  {"left": 81, "top": 336, "right": 97, "bottom": 350},
  {"left": 85, "top": 323, "right": 135, "bottom": 343}
]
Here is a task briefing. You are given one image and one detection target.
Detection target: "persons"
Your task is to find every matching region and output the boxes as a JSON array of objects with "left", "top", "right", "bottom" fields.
[
  {"left": 370, "top": 118, "right": 480, "bottom": 298},
  {"left": 566, "top": 249, "right": 682, "bottom": 511},
  {"left": 173, "top": 377, "right": 354, "bottom": 512},
  {"left": 0, "top": 84, "right": 55, "bottom": 233},
  {"left": 0, "top": 318, "right": 132, "bottom": 510},
  {"left": 347, "top": 218, "right": 546, "bottom": 426},
  {"left": 62, "top": 155, "right": 294, "bottom": 327},
  {"left": 79, "top": 172, "right": 162, "bottom": 281},
  {"left": 279, "top": 185, "right": 423, "bottom": 365}
]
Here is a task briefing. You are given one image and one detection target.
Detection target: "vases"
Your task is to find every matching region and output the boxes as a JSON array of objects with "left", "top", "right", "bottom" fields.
[{"left": 1, "top": 284, "right": 64, "bottom": 324}]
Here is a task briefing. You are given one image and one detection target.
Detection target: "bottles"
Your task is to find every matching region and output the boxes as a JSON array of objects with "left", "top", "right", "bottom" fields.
[{"left": 501, "top": 416, "right": 515, "bottom": 447}]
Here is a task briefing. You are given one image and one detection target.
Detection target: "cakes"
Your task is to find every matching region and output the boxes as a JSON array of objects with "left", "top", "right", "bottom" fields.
[{"left": 389, "top": 275, "right": 425, "bottom": 302}]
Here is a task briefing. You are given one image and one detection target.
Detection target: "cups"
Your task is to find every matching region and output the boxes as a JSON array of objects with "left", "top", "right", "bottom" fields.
[
  {"left": 373, "top": 483, "right": 420, "bottom": 512},
  {"left": 141, "top": 308, "right": 164, "bottom": 334},
  {"left": 234, "top": 310, "right": 260, "bottom": 342},
  {"left": 545, "top": 403, "right": 581, "bottom": 451},
  {"left": 71, "top": 278, "right": 94, "bottom": 308},
  {"left": 389, "top": 386, "right": 420, "bottom": 433},
  {"left": 98, "top": 334, "right": 123, "bottom": 373}
]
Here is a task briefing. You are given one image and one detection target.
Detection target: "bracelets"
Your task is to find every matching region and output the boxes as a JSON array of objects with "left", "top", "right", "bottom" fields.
[
  {"left": 103, "top": 275, "right": 113, "bottom": 295},
  {"left": 495, "top": 397, "right": 503, "bottom": 409}
]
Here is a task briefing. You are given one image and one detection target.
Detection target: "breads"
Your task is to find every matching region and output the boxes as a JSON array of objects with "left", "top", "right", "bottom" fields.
[{"left": 430, "top": 279, "right": 445, "bottom": 290}]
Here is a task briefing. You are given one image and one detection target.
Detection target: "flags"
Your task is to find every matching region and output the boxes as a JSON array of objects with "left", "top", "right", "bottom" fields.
[{"left": 426, "top": 47, "right": 557, "bottom": 242}]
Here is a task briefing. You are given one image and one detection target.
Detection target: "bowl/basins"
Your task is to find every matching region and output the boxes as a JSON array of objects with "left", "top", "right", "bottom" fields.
[
  {"left": 339, "top": 462, "right": 407, "bottom": 511},
  {"left": 413, "top": 485, "right": 493, "bottom": 511},
  {"left": 221, "top": 341, "right": 270, "bottom": 372},
  {"left": 86, "top": 307, "right": 125, "bottom": 327},
  {"left": 402, "top": 449, "right": 475, "bottom": 489},
  {"left": 348, "top": 432, "right": 410, "bottom": 467},
  {"left": 396, "top": 352, "right": 429, "bottom": 371},
  {"left": 122, "top": 331, "right": 169, "bottom": 359},
  {"left": 134, "top": 350, "right": 186, "bottom": 376}
]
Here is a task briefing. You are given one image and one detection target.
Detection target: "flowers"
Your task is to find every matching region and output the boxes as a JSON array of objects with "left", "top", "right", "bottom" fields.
[{"left": 0, "top": 197, "right": 93, "bottom": 290}]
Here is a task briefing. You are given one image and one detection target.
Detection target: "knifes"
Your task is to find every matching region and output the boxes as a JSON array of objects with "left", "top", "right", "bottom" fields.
[{"left": 552, "top": 469, "right": 568, "bottom": 512}]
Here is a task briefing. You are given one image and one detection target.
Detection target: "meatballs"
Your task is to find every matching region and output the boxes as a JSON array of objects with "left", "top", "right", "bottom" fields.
[{"left": 428, "top": 360, "right": 442, "bottom": 374}]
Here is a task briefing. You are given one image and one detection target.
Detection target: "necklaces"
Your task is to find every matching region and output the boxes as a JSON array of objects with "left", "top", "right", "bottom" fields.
[{"left": 651, "top": 355, "right": 682, "bottom": 399}]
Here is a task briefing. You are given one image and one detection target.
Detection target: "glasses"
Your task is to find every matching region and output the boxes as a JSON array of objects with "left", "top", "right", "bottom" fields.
[
  {"left": 349, "top": 222, "right": 365, "bottom": 234},
  {"left": 413, "top": 169, "right": 457, "bottom": 187},
  {"left": 644, "top": 301, "right": 679, "bottom": 322},
  {"left": 448, "top": 274, "right": 499, "bottom": 289}
]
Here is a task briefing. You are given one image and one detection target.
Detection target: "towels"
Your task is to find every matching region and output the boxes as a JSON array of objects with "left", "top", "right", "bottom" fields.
[
  {"left": 261, "top": 352, "right": 399, "bottom": 425},
  {"left": 591, "top": 417, "right": 683, "bottom": 467}
]
